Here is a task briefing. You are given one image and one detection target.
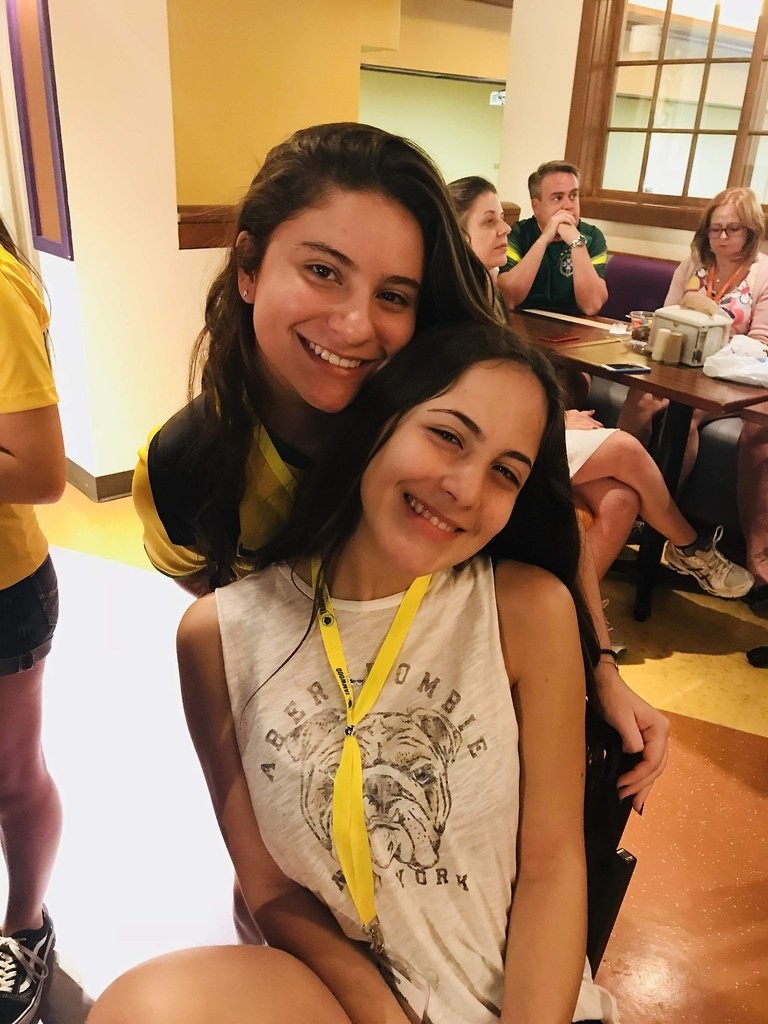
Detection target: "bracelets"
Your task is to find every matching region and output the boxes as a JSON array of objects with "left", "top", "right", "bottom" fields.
[{"left": 599, "top": 650, "right": 618, "bottom": 670}]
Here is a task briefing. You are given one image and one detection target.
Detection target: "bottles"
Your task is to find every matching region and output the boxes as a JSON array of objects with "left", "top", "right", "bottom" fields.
[
  {"left": 663, "top": 332, "right": 683, "bottom": 365},
  {"left": 651, "top": 328, "right": 670, "bottom": 360}
]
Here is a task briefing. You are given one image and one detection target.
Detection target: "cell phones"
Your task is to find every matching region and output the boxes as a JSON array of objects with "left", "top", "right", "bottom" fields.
[
  {"left": 600, "top": 362, "right": 651, "bottom": 372},
  {"left": 539, "top": 332, "right": 579, "bottom": 342}
]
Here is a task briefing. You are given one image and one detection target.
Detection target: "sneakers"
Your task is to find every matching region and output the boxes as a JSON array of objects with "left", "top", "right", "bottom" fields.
[
  {"left": 0, "top": 906, "right": 52, "bottom": 1024},
  {"left": 601, "top": 598, "right": 627, "bottom": 660},
  {"left": 664, "top": 523, "right": 755, "bottom": 599}
]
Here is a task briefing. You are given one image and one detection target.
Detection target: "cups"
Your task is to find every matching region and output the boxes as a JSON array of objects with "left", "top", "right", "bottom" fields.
[{"left": 630, "top": 311, "right": 654, "bottom": 354}]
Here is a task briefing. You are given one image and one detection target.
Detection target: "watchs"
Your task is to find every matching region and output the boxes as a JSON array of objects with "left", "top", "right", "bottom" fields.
[{"left": 568, "top": 234, "right": 585, "bottom": 249}]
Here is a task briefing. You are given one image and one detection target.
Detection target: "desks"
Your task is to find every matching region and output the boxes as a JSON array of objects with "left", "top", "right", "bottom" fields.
[{"left": 494, "top": 304, "right": 768, "bottom": 621}]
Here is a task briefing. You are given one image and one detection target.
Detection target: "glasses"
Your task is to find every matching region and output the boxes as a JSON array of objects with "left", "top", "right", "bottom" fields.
[{"left": 704, "top": 223, "right": 746, "bottom": 238}]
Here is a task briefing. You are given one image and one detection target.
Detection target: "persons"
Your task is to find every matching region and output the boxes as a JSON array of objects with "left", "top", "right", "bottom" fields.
[
  {"left": 84, "top": 323, "right": 620, "bottom": 1024},
  {"left": 494, "top": 159, "right": 608, "bottom": 411},
  {"left": 616, "top": 186, "right": 768, "bottom": 539},
  {"left": 445, "top": 176, "right": 754, "bottom": 656},
  {"left": 131, "top": 123, "right": 671, "bottom": 946},
  {"left": 0, "top": 213, "right": 67, "bottom": 1024}
]
